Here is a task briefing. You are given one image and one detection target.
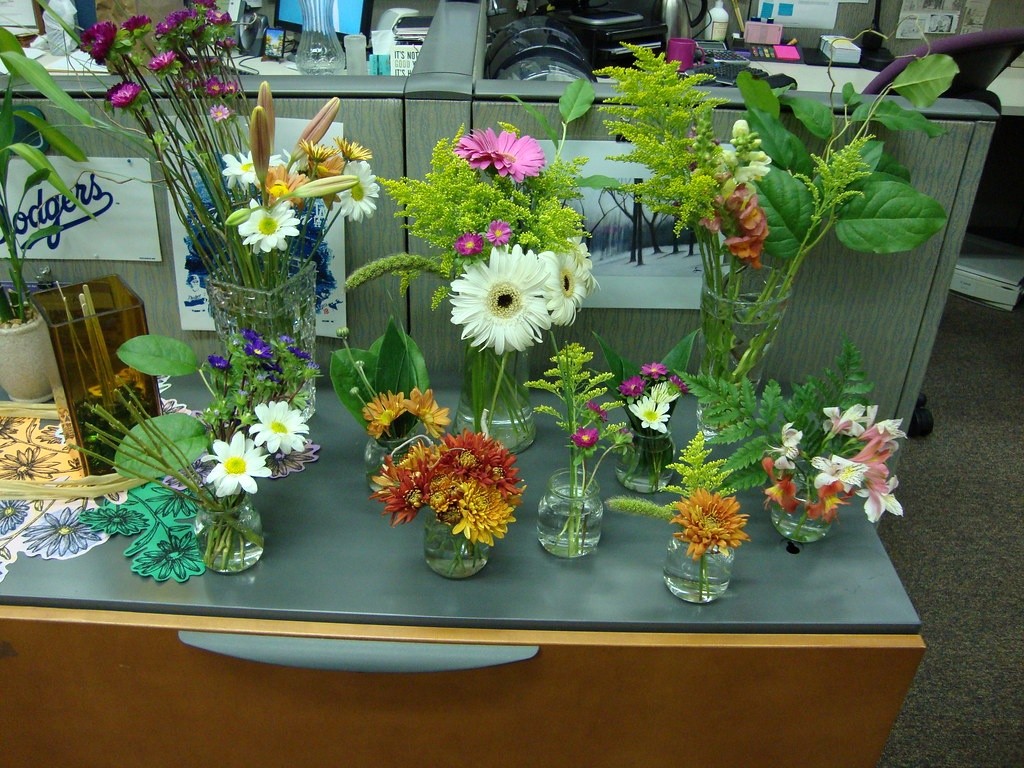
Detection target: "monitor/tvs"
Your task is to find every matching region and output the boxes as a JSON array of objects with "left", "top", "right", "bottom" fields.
[{"left": 273, "top": 0, "right": 374, "bottom": 62}]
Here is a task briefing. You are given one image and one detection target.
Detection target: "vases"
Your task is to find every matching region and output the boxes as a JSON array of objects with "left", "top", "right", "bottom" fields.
[
  {"left": 199, "top": 253, "right": 320, "bottom": 428},
  {"left": 534, "top": 468, "right": 604, "bottom": 561},
  {"left": 615, "top": 426, "right": 677, "bottom": 494},
  {"left": 693, "top": 262, "right": 796, "bottom": 444},
  {"left": 30, "top": 274, "right": 162, "bottom": 484},
  {"left": 192, "top": 488, "right": 266, "bottom": 574},
  {"left": 452, "top": 334, "right": 534, "bottom": 455},
  {"left": 772, "top": 474, "right": 833, "bottom": 543},
  {"left": 364, "top": 424, "right": 425, "bottom": 494},
  {"left": 422, "top": 507, "right": 488, "bottom": 579},
  {"left": 663, "top": 537, "right": 735, "bottom": 601},
  {"left": 295, "top": 0, "right": 346, "bottom": 75}
]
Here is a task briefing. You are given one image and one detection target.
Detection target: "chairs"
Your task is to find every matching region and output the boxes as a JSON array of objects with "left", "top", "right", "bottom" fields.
[{"left": 864, "top": 29, "right": 1024, "bottom": 119}]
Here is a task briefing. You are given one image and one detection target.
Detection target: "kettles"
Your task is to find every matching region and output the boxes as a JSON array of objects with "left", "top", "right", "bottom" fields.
[{"left": 650, "top": 0, "right": 707, "bottom": 57}]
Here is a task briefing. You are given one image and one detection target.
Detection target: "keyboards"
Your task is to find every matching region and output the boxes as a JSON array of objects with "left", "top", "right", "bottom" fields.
[{"left": 681, "top": 61, "right": 769, "bottom": 86}]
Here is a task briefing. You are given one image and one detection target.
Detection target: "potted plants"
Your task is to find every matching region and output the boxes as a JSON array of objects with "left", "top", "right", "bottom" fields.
[{"left": 0, "top": 0, "right": 106, "bottom": 404}]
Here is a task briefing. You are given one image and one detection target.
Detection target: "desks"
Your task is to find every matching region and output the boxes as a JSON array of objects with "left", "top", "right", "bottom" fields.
[{"left": 0, "top": 383, "right": 927, "bottom": 768}]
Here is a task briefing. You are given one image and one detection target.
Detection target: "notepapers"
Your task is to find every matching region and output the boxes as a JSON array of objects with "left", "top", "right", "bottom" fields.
[{"left": 773, "top": 45, "right": 800, "bottom": 60}]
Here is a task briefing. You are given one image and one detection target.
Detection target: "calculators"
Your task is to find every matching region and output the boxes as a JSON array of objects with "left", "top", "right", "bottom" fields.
[{"left": 693, "top": 40, "right": 749, "bottom": 64}]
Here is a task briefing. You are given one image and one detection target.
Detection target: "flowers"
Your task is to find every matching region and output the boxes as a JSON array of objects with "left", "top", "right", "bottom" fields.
[{"left": 64, "top": 0, "right": 961, "bottom": 582}]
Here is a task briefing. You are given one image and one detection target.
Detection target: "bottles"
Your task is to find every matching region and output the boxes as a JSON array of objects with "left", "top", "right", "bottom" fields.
[
  {"left": 704, "top": 0, "right": 729, "bottom": 40},
  {"left": 296, "top": 0, "right": 345, "bottom": 75},
  {"left": 344, "top": 35, "right": 368, "bottom": 76}
]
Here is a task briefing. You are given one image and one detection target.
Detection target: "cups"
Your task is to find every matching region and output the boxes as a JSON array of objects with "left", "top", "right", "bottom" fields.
[{"left": 667, "top": 38, "right": 705, "bottom": 72}]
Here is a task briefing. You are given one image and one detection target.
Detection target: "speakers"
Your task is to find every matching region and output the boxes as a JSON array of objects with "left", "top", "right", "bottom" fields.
[{"left": 238, "top": 14, "right": 269, "bottom": 57}]
